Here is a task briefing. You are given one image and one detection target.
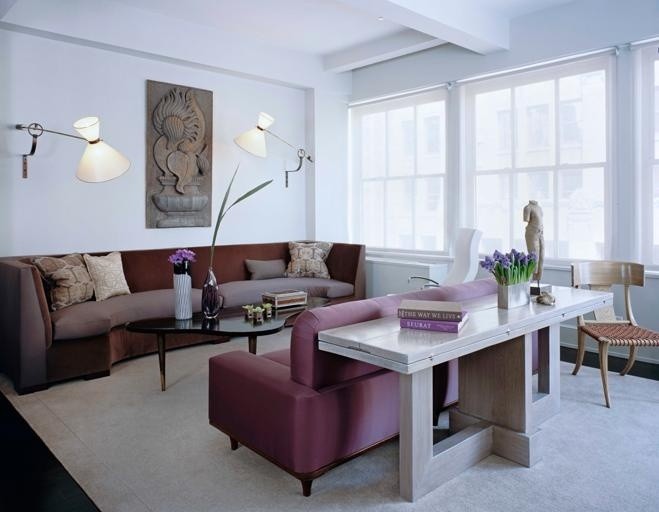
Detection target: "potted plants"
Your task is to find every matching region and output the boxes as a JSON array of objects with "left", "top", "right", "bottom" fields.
[{"left": 201, "top": 161, "right": 273, "bottom": 327}]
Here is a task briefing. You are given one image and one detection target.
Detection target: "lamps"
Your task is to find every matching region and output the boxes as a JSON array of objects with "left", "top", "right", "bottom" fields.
[
  {"left": 233, "top": 112, "right": 315, "bottom": 188},
  {"left": 15, "top": 117, "right": 131, "bottom": 182}
]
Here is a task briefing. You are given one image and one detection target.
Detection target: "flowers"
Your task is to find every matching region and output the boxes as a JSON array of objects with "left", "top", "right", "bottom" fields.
[
  {"left": 168, "top": 248, "right": 198, "bottom": 265},
  {"left": 477, "top": 248, "right": 538, "bottom": 284}
]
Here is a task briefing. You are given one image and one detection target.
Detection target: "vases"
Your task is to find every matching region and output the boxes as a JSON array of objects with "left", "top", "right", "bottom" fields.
[
  {"left": 497, "top": 282, "right": 530, "bottom": 309},
  {"left": 173, "top": 260, "right": 192, "bottom": 320}
]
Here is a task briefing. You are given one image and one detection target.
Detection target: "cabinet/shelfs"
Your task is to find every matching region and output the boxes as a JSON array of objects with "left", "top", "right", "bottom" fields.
[{"left": 366, "top": 256, "right": 448, "bottom": 298}]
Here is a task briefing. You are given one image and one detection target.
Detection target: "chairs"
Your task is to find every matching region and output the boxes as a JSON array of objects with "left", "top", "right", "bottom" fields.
[
  {"left": 572, "top": 260, "right": 659, "bottom": 408},
  {"left": 387, "top": 227, "right": 483, "bottom": 289}
]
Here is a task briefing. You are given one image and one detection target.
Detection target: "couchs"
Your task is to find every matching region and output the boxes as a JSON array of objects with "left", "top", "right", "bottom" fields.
[
  {"left": 207, "top": 276, "right": 538, "bottom": 497},
  {"left": 1, "top": 240, "right": 366, "bottom": 396}
]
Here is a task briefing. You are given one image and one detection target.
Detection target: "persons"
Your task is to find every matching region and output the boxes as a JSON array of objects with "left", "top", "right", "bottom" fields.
[{"left": 524, "top": 200, "right": 544, "bottom": 281}]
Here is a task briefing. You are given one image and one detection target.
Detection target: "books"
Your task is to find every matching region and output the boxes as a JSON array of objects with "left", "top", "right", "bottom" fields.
[
  {"left": 397, "top": 298, "right": 463, "bottom": 321},
  {"left": 399, "top": 311, "right": 469, "bottom": 333}
]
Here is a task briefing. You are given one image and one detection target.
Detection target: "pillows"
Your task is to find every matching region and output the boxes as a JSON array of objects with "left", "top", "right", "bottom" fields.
[
  {"left": 284, "top": 241, "right": 332, "bottom": 279},
  {"left": 29, "top": 253, "right": 94, "bottom": 311},
  {"left": 245, "top": 260, "right": 286, "bottom": 281},
  {"left": 81, "top": 250, "right": 131, "bottom": 301}
]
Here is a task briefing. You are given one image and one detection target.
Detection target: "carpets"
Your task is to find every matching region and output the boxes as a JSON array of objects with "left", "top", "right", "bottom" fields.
[{"left": 0, "top": 326, "right": 659, "bottom": 512}]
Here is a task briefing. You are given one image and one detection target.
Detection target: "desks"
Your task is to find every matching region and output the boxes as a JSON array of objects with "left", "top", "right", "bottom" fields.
[{"left": 317, "top": 278, "right": 615, "bottom": 503}]
